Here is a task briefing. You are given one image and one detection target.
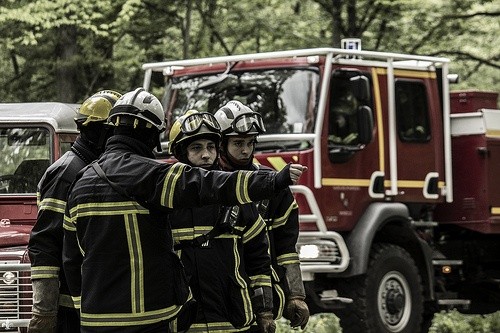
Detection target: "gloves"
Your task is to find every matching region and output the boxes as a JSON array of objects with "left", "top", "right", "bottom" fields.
[
  {"left": 284, "top": 299, "right": 310, "bottom": 329},
  {"left": 256, "top": 313, "right": 276, "bottom": 333},
  {"left": 26, "top": 315, "right": 57, "bottom": 333}
]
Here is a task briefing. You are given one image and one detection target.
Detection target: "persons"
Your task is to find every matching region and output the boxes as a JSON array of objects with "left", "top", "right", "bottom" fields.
[
  {"left": 211, "top": 99, "right": 310, "bottom": 333},
  {"left": 26, "top": 89, "right": 124, "bottom": 333},
  {"left": 60, "top": 87, "right": 308, "bottom": 333},
  {"left": 167, "top": 108, "right": 276, "bottom": 333},
  {"left": 325, "top": 91, "right": 370, "bottom": 162}
]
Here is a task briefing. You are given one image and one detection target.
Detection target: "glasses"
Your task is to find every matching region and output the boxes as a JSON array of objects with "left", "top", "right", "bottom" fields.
[
  {"left": 219, "top": 112, "right": 266, "bottom": 134},
  {"left": 170, "top": 112, "right": 221, "bottom": 148}
]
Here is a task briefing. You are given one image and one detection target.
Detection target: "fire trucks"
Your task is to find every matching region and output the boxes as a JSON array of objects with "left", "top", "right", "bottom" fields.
[
  {"left": 139, "top": 39, "right": 500, "bottom": 333},
  {"left": 0, "top": 101, "right": 84, "bottom": 333}
]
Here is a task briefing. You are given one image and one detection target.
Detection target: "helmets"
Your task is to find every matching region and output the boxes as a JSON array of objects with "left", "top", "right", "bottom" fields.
[
  {"left": 107, "top": 87, "right": 168, "bottom": 132},
  {"left": 169, "top": 110, "right": 221, "bottom": 150},
  {"left": 73, "top": 90, "right": 122, "bottom": 131},
  {"left": 214, "top": 100, "right": 266, "bottom": 140}
]
